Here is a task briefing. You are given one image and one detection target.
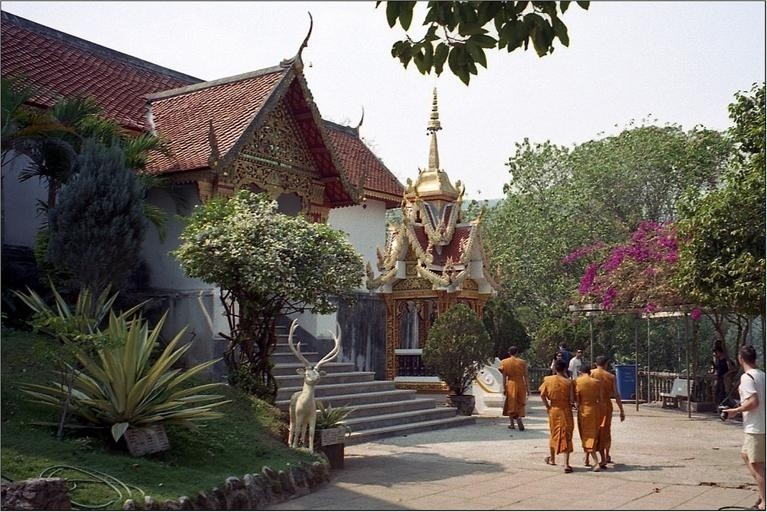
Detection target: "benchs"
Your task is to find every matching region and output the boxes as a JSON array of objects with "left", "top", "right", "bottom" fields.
[{"left": 659, "top": 378, "right": 694, "bottom": 408}]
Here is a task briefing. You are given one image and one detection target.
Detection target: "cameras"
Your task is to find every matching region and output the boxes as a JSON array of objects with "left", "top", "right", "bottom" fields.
[{"left": 717, "top": 405, "right": 730, "bottom": 421}]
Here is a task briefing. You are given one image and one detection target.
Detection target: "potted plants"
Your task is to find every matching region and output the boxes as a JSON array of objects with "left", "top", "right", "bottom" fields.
[
  {"left": 420, "top": 303, "right": 495, "bottom": 416},
  {"left": 307, "top": 399, "right": 359, "bottom": 469}
]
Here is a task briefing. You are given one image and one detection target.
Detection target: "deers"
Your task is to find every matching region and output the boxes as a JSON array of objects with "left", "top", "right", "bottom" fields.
[{"left": 288, "top": 318, "right": 342, "bottom": 454}]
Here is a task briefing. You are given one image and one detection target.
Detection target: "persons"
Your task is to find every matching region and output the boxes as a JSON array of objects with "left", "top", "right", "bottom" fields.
[
  {"left": 497, "top": 345, "right": 530, "bottom": 431},
  {"left": 711, "top": 345, "right": 728, "bottom": 416},
  {"left": 721, "top": 345, "right": 766, "bottom": 511},
  {"left": 538, "top": 341, "right": 626, "bottom": 473}
]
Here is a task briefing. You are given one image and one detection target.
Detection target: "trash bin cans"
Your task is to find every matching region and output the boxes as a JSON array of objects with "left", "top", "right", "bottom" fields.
[{"left": 614, "top": 365, "right": 636, "bottom": 400}]
